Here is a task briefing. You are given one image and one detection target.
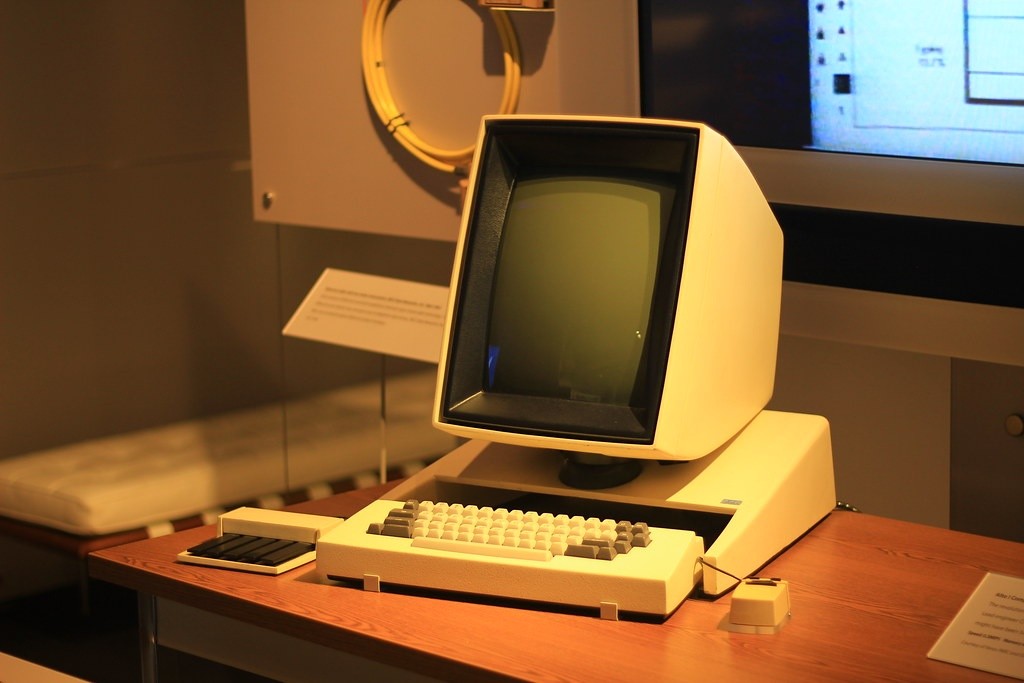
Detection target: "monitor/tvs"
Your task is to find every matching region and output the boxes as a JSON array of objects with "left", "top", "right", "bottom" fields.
[
  {"left": 431, "top": 113, "right": 784, "bottom": 462},
  {"left": 624, "top": 1, "right": 1024, "bottom": 228}
]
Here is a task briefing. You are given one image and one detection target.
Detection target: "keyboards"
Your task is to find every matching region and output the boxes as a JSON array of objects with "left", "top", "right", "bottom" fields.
[{"left": 318, "top": 499, "right": 706, "bottom": 617}]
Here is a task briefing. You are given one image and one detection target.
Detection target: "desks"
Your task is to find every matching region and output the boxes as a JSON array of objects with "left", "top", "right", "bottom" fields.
[{"left": 85, "top": 474, "right": 1024, "bottom": 683}]
